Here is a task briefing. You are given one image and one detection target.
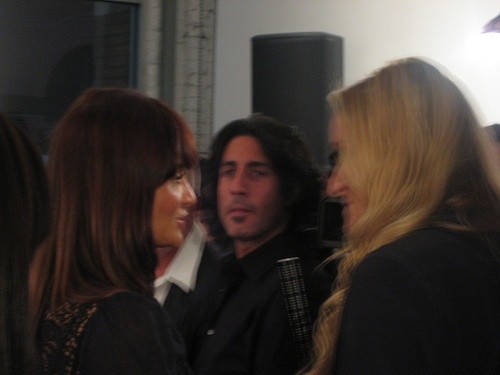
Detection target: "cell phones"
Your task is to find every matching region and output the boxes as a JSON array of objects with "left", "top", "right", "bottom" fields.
[{"left": 316, "top": 194, "right": 345, "bottom": 249}]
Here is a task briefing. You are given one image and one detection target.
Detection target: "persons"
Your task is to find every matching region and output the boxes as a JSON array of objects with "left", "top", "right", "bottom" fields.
[
  {"left": 200, "top": 113, "right": 339, "bottom": 372},
  {"left": 482, "top": 119, "right": 500, "bottom": 155},
  {"left": 2, "top": 114, "right": 351, "bottom": 293},
  {"left": 314, "top": 56, "right": 500, "bottom": 375},
  {"left": 11, "top": 84, "right": 203, "bottom": 374}
]
